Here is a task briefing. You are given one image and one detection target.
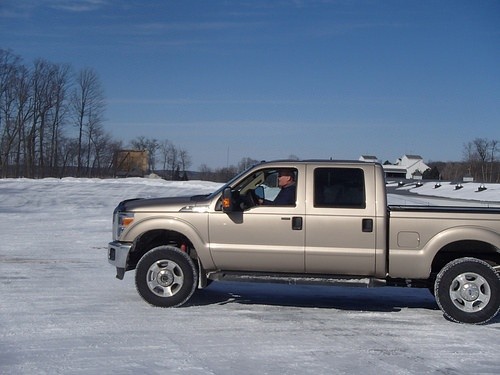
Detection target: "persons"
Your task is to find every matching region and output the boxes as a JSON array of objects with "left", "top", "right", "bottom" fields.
[{"left": 257, "top": 168, "right": 296, "bottom": 206}]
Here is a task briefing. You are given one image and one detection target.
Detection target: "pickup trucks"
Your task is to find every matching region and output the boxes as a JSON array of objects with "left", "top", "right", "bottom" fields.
[{"left": 108, "top": 158, "right": 499, "bottom": 325}]
